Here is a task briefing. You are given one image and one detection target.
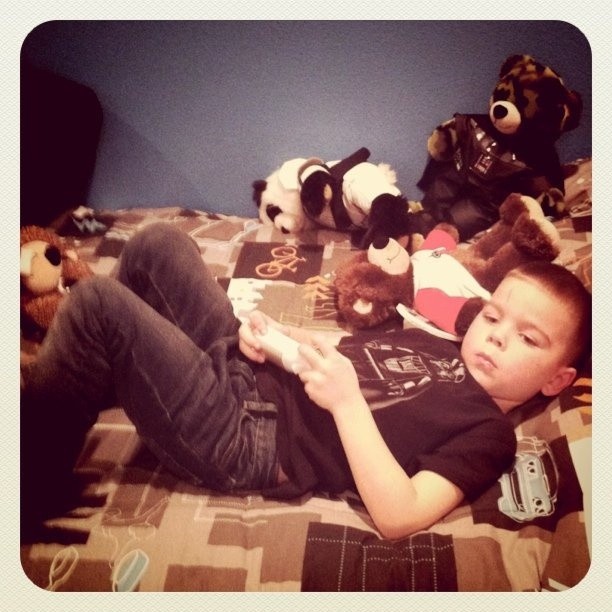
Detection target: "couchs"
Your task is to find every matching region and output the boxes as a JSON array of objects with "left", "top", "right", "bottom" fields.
[{"left": 20, "top": 74, "right": 106, "bottom": 228}]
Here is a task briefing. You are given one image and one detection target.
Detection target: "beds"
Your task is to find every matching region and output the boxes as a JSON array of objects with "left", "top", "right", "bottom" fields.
[{"left": 22, "top": 198, "right": 590, "bottom": 591}]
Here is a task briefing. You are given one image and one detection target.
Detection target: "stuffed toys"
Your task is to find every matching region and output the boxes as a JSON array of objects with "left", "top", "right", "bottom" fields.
[
  {"left": 20, "top": 225, "right": 96, "bottom": 330},
  {"left": 331, "top": 192, "right": 564, "bottom": 337},
  {"left": 249, "top": 146, "right": 410, "bottom": 239},
  {"left": 411, "top": 53, "right": 584, "bottom": 242}
]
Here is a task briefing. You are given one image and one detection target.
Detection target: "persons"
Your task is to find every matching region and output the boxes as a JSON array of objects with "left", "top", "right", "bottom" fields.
[{"left": 20, "top": 220, "right": 592, "bottom": 541}]
